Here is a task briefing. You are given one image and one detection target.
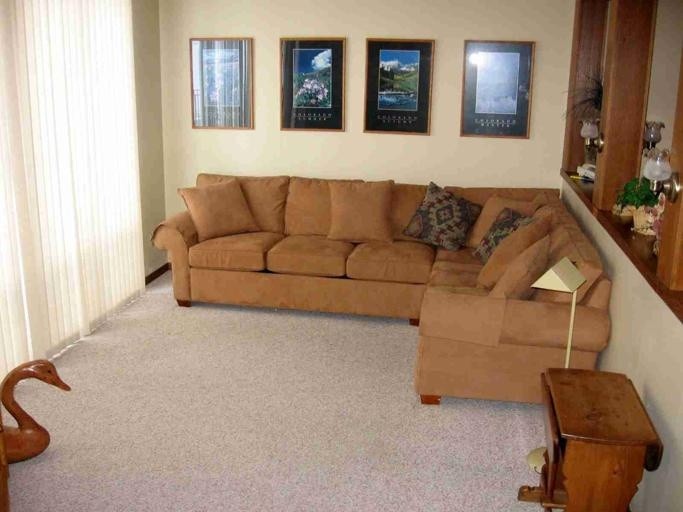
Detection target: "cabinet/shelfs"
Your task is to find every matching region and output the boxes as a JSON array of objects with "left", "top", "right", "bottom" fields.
[{"left": 517, "top": 367, "right": 664, "bottom": 512}]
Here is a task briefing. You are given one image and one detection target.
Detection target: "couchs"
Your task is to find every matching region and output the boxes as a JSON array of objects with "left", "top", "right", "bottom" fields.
[{"left": 149, "top": 174, "right": 610, "bottom": 405}]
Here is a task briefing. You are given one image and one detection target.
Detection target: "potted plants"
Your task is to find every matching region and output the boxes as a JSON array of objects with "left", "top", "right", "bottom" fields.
[{"left": 615, "top": 178, "right": 656, "bottom": 236}]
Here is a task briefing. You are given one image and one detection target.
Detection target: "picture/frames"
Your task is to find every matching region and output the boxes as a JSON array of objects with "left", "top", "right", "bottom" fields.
[
  {"left": 280, "top": 36, "right": 346, "bottom": 132},
  {"left": 361, "top": 38, "right": 436, "bottom": 135},
  {"left": 460, "top": 39, "right": 536, "bottom": 140},
  {"left": 189, "top": 38, "right": 254, "bottom": 130}
]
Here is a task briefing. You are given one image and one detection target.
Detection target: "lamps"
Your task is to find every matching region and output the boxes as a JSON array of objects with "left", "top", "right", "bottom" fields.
[
  {"left": 644, "top": 121, "right": 666, "bottom": 148},
  {"left": 644, "top": 147, "right": 672, "bottom": 192},
  {"left": 529, "top": 257, "right": 588, "bottom": 369},
  {"left": 580, "top": 119, "right": 600, "bottom": 151}
]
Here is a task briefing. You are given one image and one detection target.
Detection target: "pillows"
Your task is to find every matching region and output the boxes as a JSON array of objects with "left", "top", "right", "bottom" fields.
[
  {"left": 488, "top": 237, "right": 549, "bottom": 302},
  {"left": 326, "top": 180, "right": 393, "bottom": 245},
  {"left": 176, "top": 176, "right": 262, "bottom": 242},
  {"left": 401, "top": 181, "right": 483, "bottom": 252},
  {"left": 472, "top": 207, "right": 535, "bottom": 263},
  {"left": 462, "top": 194, "right": 529, "bottom": 248},
  {"left": 477, "top": 213, "right": 552, "bottom": 290}
]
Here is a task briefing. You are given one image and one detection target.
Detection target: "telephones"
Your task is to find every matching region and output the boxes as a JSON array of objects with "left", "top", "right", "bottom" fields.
[{"left": 576, "top": 163, "right": 596, "bottom": 179}]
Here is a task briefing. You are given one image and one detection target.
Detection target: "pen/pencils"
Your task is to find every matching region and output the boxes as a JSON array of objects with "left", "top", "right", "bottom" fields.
[{"left": 570, "top": 176, "right": 591, "bottom": 181}]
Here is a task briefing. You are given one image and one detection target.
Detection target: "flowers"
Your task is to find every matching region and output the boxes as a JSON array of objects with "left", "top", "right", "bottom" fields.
[{"left": 630, "top": 192, "right": 666, "bottom": 240}]
